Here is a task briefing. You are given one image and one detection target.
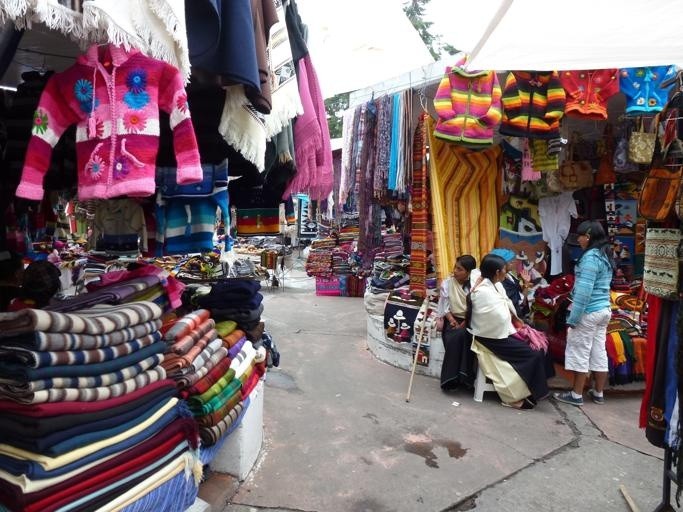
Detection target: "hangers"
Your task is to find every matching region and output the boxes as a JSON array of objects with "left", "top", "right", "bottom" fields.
[
  {"left": 87, "top": 25, "right": 112, "bottom": 53},
  {"left": 36, "top": 63, "right": 47, "bottom": 77}
]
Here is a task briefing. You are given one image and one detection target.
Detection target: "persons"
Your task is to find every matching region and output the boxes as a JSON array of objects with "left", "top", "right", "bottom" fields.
[
  {"left": 464, "top": 253, "right": 551, "bottom": 410},
  {"left": 0, "top": 259, "right": 26, "bottom": 311},
  {"left": 437, "top": 255, "right": 477, "bottom": 393},
  {"left": 490, "top": 247, "right": 556, "bottom": 380},
  {"left": 0, "top": 259, "right": 61, "bottom": 311},
  {"left": 552, "top": 217, "right": 617, "bottom": 406}
]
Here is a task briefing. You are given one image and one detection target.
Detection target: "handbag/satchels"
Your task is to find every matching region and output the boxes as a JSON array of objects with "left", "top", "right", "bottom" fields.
[
  {"left": 502, "top": 153, "right": 562, "bottom": 211},
  {"left": 641, "top": 226, "right": 681, "bottom": 300},
  {"left": 628, "top": 91, "right": 683, "bottom": 221},
  {"left": 557, "top": 115, "right": 639, "bottom": 190}
]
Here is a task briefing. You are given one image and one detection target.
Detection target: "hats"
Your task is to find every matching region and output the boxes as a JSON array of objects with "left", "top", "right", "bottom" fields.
[
  {"left": 400, "top": 322, "right": 411, "bottom": 330},
  {"left": 392, "top": 310, "right": 406, "bottom": 320},
  {"left": 388, "top": 318, "right": 396, "bottom": 327},
  {"left": 490, "top": 248, "right": 516, "bottom": 262}
]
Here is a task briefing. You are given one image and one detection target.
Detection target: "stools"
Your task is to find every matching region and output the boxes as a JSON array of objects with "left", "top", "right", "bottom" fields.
[{"left": 472, "top": 359, "right": 500, "bottom": 404}]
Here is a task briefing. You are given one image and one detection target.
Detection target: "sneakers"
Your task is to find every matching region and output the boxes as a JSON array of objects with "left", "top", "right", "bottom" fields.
[
  {"left": 553, "top": 391, "right": 583, "bottom": 406},
  {"left": 586, "top": 389, "right": 603, "bottom": 404}
]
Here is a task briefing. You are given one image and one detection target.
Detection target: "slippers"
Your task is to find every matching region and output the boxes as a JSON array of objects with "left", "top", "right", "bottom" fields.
[
  {"left": 525, "top": 396, "right": 537, "bottom": 405},
  {"left": 501, "top": 400, "right": 533, "bottom": 410}
]
